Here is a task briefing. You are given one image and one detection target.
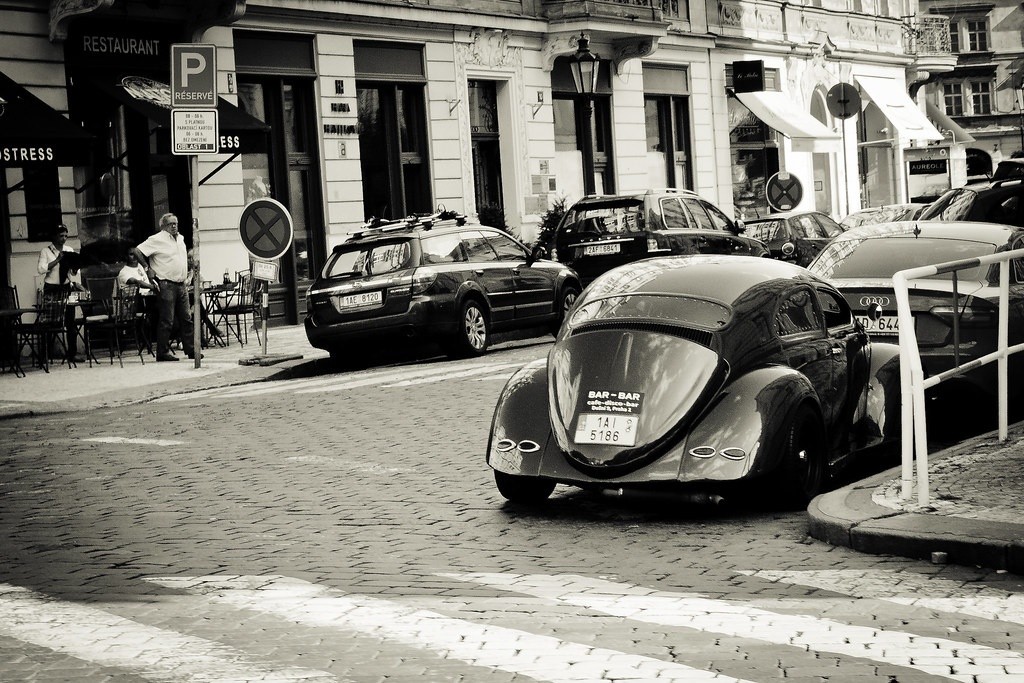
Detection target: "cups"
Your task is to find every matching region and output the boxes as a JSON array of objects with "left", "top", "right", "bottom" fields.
[{"left": 69, "top": 296, "right": 78, "bottom": 301}]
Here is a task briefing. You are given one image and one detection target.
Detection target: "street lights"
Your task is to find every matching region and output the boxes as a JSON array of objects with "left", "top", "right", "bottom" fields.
[{"left": 570, "top": 31, "right": 602, "bottom": 201}]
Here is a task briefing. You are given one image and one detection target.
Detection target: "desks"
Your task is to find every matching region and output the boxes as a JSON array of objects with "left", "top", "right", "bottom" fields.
[
  {"left": 188, "top": 288, "right": 245, "bottom": 348},
  {"left": 112, "top": 295, "right": 177, "bottom": 356},
  {"left": 31, "top": 300, "right": 100, "bottom": 364}
]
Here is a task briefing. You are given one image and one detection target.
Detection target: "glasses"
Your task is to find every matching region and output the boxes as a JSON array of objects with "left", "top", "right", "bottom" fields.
[{"left": 58, "top": 224, "right": 66, "bottom": 228}]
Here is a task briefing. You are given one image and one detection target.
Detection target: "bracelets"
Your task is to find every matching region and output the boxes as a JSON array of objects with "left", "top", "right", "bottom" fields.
[{"left": 144, "top": 267, "right": 150, "bottom": 271}]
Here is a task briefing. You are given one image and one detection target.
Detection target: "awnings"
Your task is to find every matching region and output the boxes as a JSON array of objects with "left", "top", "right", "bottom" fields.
[
  {"left": 855, "top": 76, "right": 944, "bottom": 145},
  {"left": 100, "top": 72, "right": 272, "bottom": 156},
  {"left": 925, "top": 97, "right": 976, "bottom": 144},
  {"left": 0, "top": 72, "right": 98, "bottom": 168},
  {"left": 728, "top": 90, "right": 842, "bottom": 153}
]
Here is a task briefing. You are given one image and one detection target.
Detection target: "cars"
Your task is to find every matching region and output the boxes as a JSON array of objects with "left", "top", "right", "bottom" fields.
[
  {"left": 918, "top": 157, "right": 1024, "bottom": 227},
  {"left": 803, "top": 221, "right": 1024, "bottom": 424},
  {"left": 741, "top": 208, "right": 848, "bottom": 269},
  {"left": 485, "top": 254, "right": 899, "bottom": 507},
  {"left": 837, "top": 203, "right": 935, "bottom": 232}
]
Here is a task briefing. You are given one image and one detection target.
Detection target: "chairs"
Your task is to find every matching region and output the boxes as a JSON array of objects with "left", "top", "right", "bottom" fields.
[{"left": 0, "top": 268, "right": 268, "bottom": 378}]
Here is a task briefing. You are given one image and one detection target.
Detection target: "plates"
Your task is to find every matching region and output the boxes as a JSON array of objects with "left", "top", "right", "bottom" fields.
[{"left": 68, "top": 301, "right": 79, "bottom": 303}]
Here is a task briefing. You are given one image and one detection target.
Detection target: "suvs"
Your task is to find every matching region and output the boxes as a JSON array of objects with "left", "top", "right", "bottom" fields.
[
  {"left": 551, "top": 187, "right": 771, "bottom": 291},
  {"left": 304, "top": 208, "right": 583, "bottom": 370}
]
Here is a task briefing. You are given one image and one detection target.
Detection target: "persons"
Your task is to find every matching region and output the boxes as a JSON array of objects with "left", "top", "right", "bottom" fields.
[
  {"left": 37, "top": 225, "right": 85, "bottom": 363},
  {"left": 112, "top": 247, "right": 154, "bottom": 317},
  {"left": 133, "top": 213, "right": 204, "bottom": 361},
  {"left": 186, "top": 249, "right": 224, "bottom": 347}
]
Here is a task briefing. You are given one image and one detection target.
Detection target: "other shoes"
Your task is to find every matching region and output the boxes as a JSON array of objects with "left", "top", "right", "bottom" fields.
[
  {"left": 188, "top": 352, "right": 205, "bottom": 359},
  {"left": 155, "top": 352, "right": 178, "bottom": 361}
]
